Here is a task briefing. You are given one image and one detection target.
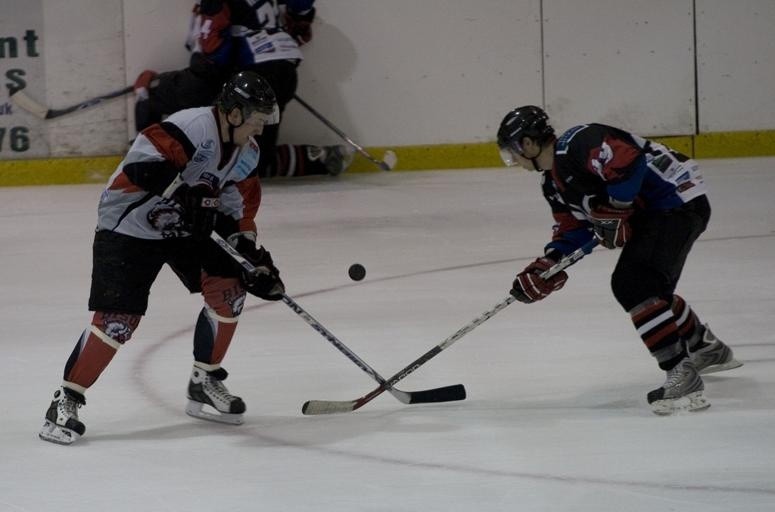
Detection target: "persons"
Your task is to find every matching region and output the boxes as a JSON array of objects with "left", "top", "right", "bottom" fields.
[
  {"left": 45, "top": 72, "right": 285, "bottom": 438},
  {"left": 134, "top": 0, "right": 346, "bottom": 179},
  {"left": 497, "top": 106, "right": 733, "bottom": 404}
]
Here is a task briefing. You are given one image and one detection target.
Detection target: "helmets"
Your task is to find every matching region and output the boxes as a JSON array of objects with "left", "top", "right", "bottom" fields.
[
  {"left": 217, "top": 71, "right": 276, "bottom": 118},
  {"left": 497, "top": 105, "right": 549, "bottom": 155}
]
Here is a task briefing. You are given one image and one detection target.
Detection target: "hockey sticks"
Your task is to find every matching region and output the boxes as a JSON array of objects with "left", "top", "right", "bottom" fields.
[
  {"left": 210, "top": 230, "right": 466, "bottom": 404},
  {"left": 302, "top": 234, "right": 601, "bottom": 415},
  {"left": 293, "top": 94, "right": 398, "bottom": 170},
  {"left": 9, "top": 86, "right": 134, "bottom": 123}
]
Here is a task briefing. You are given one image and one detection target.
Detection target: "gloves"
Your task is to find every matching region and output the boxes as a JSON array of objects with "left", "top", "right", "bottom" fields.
[
  {"left": 239, "top": 264, "right": 286, "bottom": 301},
  {"left": 587, "top": 206, "right": 632, "bottom": 250},
  {"left": 510, "top": 257, "right": 569, "bottom": 304},
  {"left": 133, "top": 70, "right": 159, "bottom": 100},
  {"left": 169, "top": 182, "right": 220, "bottom": 233}
]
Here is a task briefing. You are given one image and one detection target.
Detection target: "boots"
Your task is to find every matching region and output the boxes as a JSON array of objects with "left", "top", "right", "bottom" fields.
[
  {"left": 646, "top": 323, "right": 733, "bottom": 404},
  {"left": 186, "top": 365, "right": 246, "bottom": 414},
  {"left": 313, "top": 142, "right": 344, "bottom": 176},
  {"left": 46, "top": 390, "right": 86, "bottom": 436}
]
